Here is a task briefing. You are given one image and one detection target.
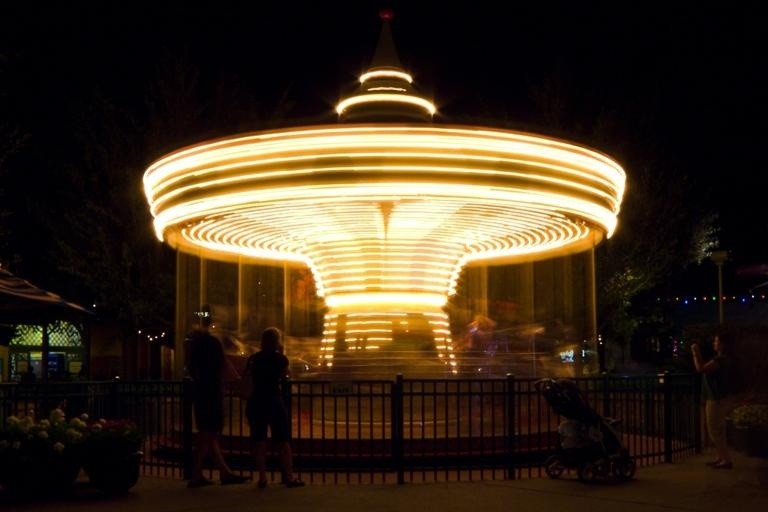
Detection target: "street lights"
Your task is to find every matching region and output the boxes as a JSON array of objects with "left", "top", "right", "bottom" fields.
[{"left": 707, "top": 250, "right": 728, "bottom": 321}]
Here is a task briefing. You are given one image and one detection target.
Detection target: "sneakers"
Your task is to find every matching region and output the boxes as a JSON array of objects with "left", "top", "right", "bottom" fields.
[
  {"left": 188, "top": 478, "right": 215, "bottom": 487},
  {"left": 222, "top": 474, "right": 250, "bottom": 484},
  {"left": 708, "top": 458, "right": 732, "bottom": 469}
]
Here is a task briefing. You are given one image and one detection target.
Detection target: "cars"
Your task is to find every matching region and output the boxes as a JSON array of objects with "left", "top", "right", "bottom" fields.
[{"left": 209, "top": 332, "right": 312, "bottom": 392}]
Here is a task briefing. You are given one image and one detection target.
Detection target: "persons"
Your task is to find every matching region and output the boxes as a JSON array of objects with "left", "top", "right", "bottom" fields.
[
  {"left": 228, "top": 327, "right": 308, "bottom": 488},
  {"left": 183, "top": 303, "right": 255, "bottom": 488},
  {"left": 690, "top": 328, "right": 737, "bottom": 469}
]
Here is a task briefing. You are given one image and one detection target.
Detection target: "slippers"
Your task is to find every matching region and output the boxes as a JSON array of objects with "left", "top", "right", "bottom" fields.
[
  {"left": 287, "top": 478, "right": 305, "bottom": 487},
  {"left": 257, "top": 480, "right": 267, "bottom": 488}
]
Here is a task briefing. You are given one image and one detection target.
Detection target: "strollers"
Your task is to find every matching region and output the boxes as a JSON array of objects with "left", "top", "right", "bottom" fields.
[{"left": 532, "top": 376, "right": 638, "bottom": 481}]
